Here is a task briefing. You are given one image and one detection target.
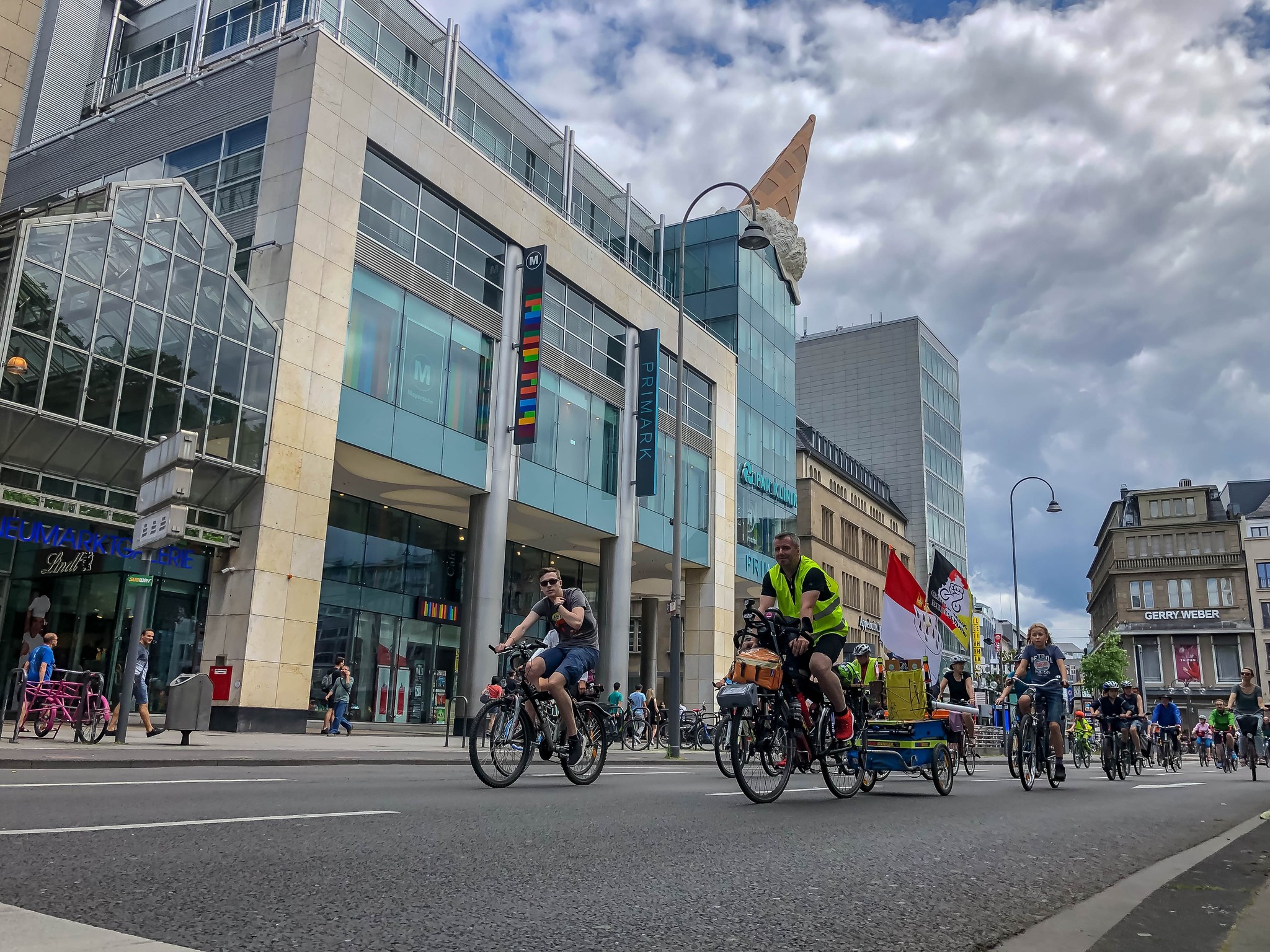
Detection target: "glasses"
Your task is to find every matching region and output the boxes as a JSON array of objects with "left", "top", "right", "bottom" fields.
[
  {"left": 540, "top": 578, "right": 560, "bottom": 587},
  {"left": 1240, "top": 672, "right": 1251, "bottom": 676}
]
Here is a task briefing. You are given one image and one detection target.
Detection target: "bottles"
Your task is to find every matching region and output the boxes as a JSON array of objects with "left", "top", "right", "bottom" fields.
[
  {"left": 789, "top": 697, "right": 802, "bottom": 721},
  {"left": 810, "top": 703, "right": 821, "bottom": 728}
]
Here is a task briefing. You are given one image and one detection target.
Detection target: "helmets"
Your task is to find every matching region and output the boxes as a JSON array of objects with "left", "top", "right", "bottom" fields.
[
  {"left": 852, "top": 644, "right": 871, "bottom": 656},
  {"left": 1011, "top": 654, "right": 1022, "bottom": 662},
  {"left": 1103, "top": 680, "right": 1132, "bottom": 689},
  {"left": 1075, "top": 710, "right": 1084, "bottom": 718},
  {"left": 949, "top": 654, "right": 968, "bottom": 665},
  {"left": 1199, "top": 714, "right": 1207, "bottom": 720}
]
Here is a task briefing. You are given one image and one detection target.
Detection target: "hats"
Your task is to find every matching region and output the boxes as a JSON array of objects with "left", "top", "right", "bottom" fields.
[
  {"left": 1158, "top": 694, "right": 1172, "bottom": 701},
  {"left": 28, "top": 595, "right": 51, "bottom": 619}
]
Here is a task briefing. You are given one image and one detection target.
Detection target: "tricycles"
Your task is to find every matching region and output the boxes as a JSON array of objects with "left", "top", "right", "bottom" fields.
[{"left": 851, "top": 696, "right": 979, "bottom": 796}]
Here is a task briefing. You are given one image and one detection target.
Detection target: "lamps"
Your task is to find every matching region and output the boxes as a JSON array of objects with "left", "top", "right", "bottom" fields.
[
  {"left": 128, "top": 610, "right": 134, "bottom": 618},
  {"left": 5, "top": 345, "right": 28, "bottom": 374},
  {"left": 517, "top": 549, "right": 523, "bottom": 556},
  {"left": 175, "top": 616, "right": 184, "bottom": 625},
  {"left": 460, "top": 530, "right": 466, "bottom": 541},
  {"left": 551, "top": 559, "right": 559, "bottom": 564},
  {"left": 96, "top": 611, "right": 103, "bottom": 618}
]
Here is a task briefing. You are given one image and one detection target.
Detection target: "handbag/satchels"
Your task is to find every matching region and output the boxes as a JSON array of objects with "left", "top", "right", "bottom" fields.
[
  {"left": 716, "top": 646, "right": 782, "bottom": 708},
  {"left": 120, "top": 673, "right": 144, "bottom": 701},
  {"left": 480, "top": 684, "right": 491, "bottom": 704},
  {"left": 349, "top": 694, "right": 354, "bottom": 706}
]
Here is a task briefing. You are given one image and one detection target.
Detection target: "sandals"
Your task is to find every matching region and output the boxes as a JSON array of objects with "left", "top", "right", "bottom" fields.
[{"left": 18, "top": 725, "right": 58, "bottom": 733}]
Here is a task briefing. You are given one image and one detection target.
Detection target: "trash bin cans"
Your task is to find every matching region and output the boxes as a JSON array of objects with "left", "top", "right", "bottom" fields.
[{"left": 163, "top": 673, "right": 214, "bottom": 732}]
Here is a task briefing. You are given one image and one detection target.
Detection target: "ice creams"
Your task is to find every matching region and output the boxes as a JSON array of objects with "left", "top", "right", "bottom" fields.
[{"left": 713, "top": 114, "right": 816, "bottom": 305}]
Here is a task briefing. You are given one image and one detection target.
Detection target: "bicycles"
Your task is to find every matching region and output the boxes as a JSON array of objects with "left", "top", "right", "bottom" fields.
[
  {"left": 33, "top": 664, "right": 111, "bottom": 745},
  {"left": 1227, "top": 707, "right": 1268, "bottom": 782},
  {"left": 489, "top": 598, "right": 978, "bottom": 780},
  {"left": 1191, "top": 730, "right": 1213, "bottom": 767},
  {"left": 1065, "top": 729, "right": 1093, "bottom": 769},
  {"left": 468, "top": 638, "right": 612, "bottom": 789},
  {"left": 1151, "top": 724, "right": 1182, "bottom": 772},
  {"left": 1210, "top": 728, "right": 1239, "bottom": 773},
  {"left": 715, "top": 598, "right": 870, "bottom": 804},
  {"left": 1006, "top": 676, "right": 1070, "bottom": 790},
  {"left": 994, "top": 699, "right": 1270, "bottom": 777}
]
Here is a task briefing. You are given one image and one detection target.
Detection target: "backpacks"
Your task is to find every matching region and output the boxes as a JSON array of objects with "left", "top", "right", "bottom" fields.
[{"left": 321, "top": 669, "right": 339, "bottom": 693}]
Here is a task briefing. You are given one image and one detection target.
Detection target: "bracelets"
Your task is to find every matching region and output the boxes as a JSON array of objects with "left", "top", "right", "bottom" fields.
[
  {"left": 999, "top": 696, "right": 1004, "bottom": 699},
  {"left": 1063, "top": 679, "right": 1068, "bottom": 682},
  {"left": 554, "top": 603, "right": 563, "bottom": 612},
  {"left": 659, "top": 716, "right": 661, "bottom": 717}
]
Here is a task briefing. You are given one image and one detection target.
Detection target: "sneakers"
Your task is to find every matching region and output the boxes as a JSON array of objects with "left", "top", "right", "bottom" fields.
[
  {"left": 1207, "top": 751, "right": 1267, "bottom": 769},
  {"left": 511, "top": 729, "right": 587, "bottom": 767},
  {"left": 834, "top": 708, "right": 854, "bottom": 742},
  {"left": 774, "top": 755, "right": 796, "bottom": 774}
]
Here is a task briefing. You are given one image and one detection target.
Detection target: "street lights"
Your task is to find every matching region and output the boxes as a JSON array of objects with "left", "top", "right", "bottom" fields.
[
  {"left": 983, "top": 637, "right": 1008, "bottom": 757},
  {"left": 1010, "top": 477, "right": 1062, "bottom": 670},
  {"left": 668, "top": 184, "right": 771, "bottom": 750}
]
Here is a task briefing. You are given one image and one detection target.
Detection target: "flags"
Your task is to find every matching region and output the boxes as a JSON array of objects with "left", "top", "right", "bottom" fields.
[
  {"left": 879, "top": 550, "right": 943, "bottom": 687},
  {"left": 927, "top": 548, "right": 973, "bottom": 650}
]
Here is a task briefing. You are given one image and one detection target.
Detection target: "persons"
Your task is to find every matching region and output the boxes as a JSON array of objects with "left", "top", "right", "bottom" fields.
[
  {"left": 741, "top": 531, "right": 855, "bottom": 767},
  {"left": 105, "top": 628, "right": 167, "bottom": 738},
  {"left": 1087, "top": 680, "right": 1182, "bottom": 772},
  {"left": 994, "top": 622, "right": 1070, "bottom": 780},
  {"left": 715, "top": 659, "right": 771, "bottom": 762},
  {"left": 493, "top": 567, "right": 600, "bottom": 767},
  {"left": 607, "top": 682, "right": 669, "bottom": 745},
  {"left": 1183, "top": 654, "right": 1200, "bottom": 678},
  {"left": 1208, "top": 699, "right": 1237, "bottom": 769},
  {"left": 1192, "top": 714, "right": 1212, "bottom": 761},
  {"left": 1262, "top": 716, "right": 1270, "bottom": 760},
  {"left": 937, "top": 654, "right": 977, "bottom": 766},
  {"left": 321, "top": 656, "right": 354, "bottom": 737},
  {"left": 1066, "top": 710, "right": 1093, "bottom": 758},
  {"left": 1224, "top": 667, "right": 1268, "bottom": 767},
  {"left": 679, "top": 700, "right": 688, "bottom": 722},
  {"left": 850, "top": 642, "right": 879, "bottom": 714},
  {"left": 501, "top": 669, "right": 520, "bottom": 699},
  {"left": 525, "top": 627, "right": 589, "bottom": 732},
  {"left": 16, "top": 595, "right": 52, "bottom": 712},
  {"left": 481, "top": 676, "right": 502, "bottom": 735},
  {"left": 17, "top": 632, "right": 59, "bottom": 733}
]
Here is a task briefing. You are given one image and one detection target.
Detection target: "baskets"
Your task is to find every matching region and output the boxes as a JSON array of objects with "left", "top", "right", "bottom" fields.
[{"left": 1236, "top": 716, "right": 1258, "bottom": 736}]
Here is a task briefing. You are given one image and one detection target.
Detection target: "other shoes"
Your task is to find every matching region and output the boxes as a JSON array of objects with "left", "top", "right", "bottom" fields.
[
  {"left": 320, "top": 728, "right": 340, "bottom": 735},
  {"left": 610, "top": 734, "right": 655, "bottom": 747},
  {"left": 968, "top": 737, "right": 976, "bottom": 747},
  {"left": 1135, "top": 752, "right": 1144, "bottom": 759},
  {"left": 104, "top": 726, "right": 167, "bottom": 738},
  {"left": 1054, "top": 763, "right": 1066, "bottom": 780},
  {"left": 327, "top": 725, "right": 354, "bottom": 736}
]
